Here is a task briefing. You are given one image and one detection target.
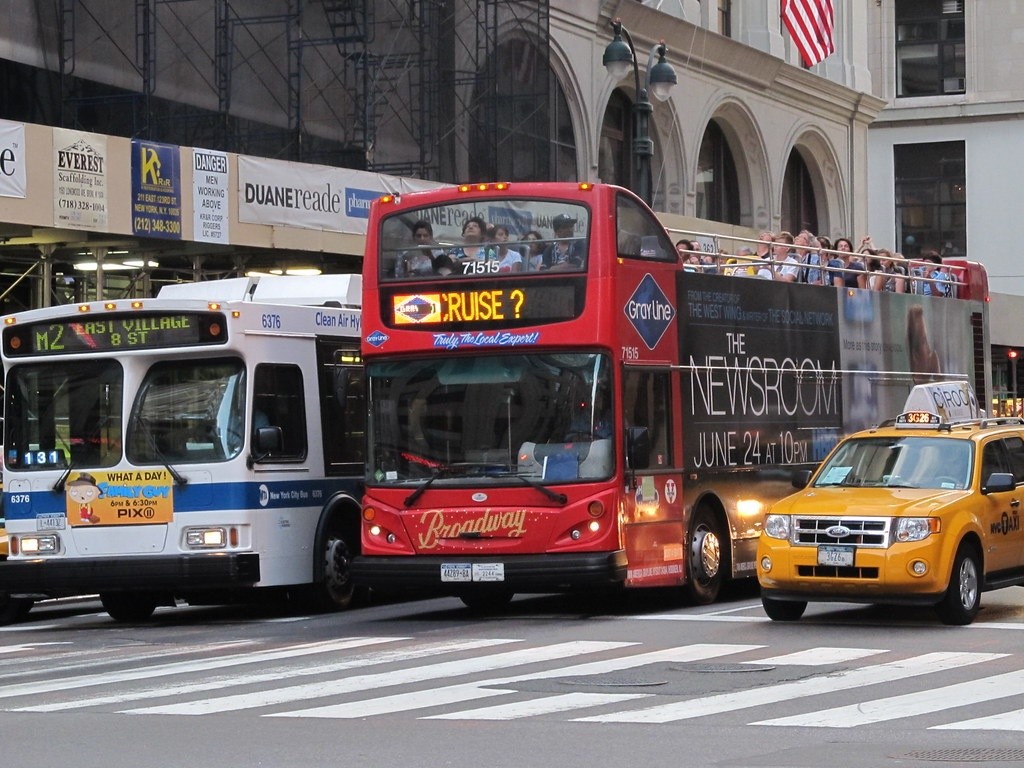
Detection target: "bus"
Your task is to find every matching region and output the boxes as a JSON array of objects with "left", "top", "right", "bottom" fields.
[
  {"left": 0, "top": 271, "right": 460, "bottom": 625},
  {"left": 349, "top": 179, "right": 992, "bottom": 620}
]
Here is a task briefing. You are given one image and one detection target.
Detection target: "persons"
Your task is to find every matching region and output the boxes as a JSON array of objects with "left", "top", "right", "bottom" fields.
[
  {"left": 906, "top": 304, "right": 941, "bottom": 396},
  {"left": 933, "top": 449, "right": 969, "bottom": 491},
  {"left": 399, "top": 212, "right": 585, "bottom": 276},
  {"left": 562, "top": 407, "right": 611, "bottom": 440},
  {"left": 675, "top": 228, "right": 953, "bottom": 297},
  {"left": 212, "top": 383, "right": 271, "bottom": 456}
]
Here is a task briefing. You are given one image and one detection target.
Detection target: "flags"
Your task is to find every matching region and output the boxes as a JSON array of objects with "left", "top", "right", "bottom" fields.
[{"left": 780, "top": 0, "right": 834, "bottom": 65}]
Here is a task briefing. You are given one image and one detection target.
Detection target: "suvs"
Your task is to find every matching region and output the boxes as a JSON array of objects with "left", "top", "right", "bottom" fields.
[{"left": 754, "top": 381, "right": 1024, "bottom": 626}]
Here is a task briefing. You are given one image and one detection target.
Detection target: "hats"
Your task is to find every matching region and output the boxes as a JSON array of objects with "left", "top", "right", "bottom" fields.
[{"left": 553, "top": 213, "right": 577, "bottom": 231}]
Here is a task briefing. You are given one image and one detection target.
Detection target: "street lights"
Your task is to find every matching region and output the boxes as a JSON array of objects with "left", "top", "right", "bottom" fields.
[{"left": 606, "top": 19, "right": 679, "bottom": 210}]
